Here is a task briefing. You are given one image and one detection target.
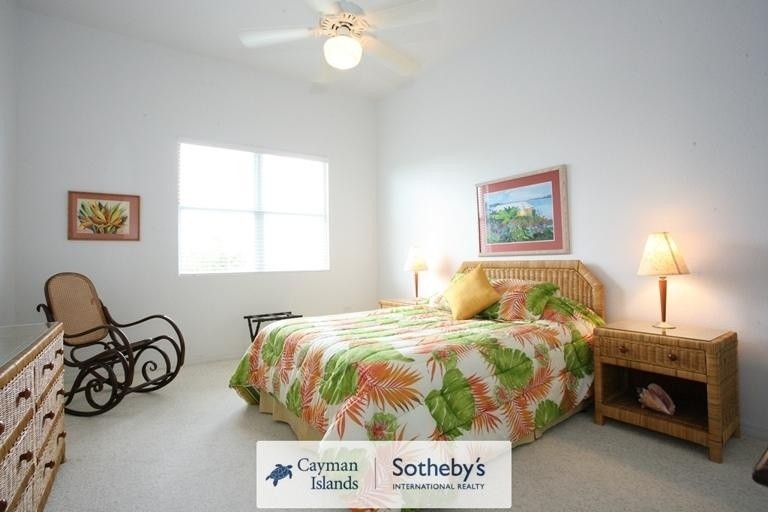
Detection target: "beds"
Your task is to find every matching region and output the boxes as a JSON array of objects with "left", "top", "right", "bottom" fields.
[{"left": 229, "top": 260, "right": 604, "bottom": 512}]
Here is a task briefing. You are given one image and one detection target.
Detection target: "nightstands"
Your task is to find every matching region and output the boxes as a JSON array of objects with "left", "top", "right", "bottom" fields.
[
  {"left": 378, "top": 296, "right": 438, "bottom": 309},
  {"left": 593, "top": 318, "right": 741, "bottom": 463}
]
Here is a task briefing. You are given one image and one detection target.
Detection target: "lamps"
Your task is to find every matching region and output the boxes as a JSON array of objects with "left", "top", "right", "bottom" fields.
[
  {"left": 636, "top": 232, "right": 691, "bottom": 329},
  {"left": 323, "top": 26, "right": 363, "bottom": 71},
  {"left": 405, "top": 248, "right": 429, "bottom": 305}
]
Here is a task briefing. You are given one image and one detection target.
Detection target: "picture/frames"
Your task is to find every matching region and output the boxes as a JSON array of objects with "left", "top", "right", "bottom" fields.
[
  {"left": 473, "top": 164, "right": 571, "bottom": 257},
  {"left": 68, "top": 191, "right": 140, "bottom": 240}
]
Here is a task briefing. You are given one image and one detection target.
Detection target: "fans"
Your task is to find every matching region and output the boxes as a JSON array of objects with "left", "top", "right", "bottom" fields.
[{"left": 239, "top": 0, "right": 441, "bottom": 78}]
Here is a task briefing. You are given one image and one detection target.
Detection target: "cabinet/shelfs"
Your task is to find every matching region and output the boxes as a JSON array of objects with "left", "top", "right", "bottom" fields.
[{"left": 0, "top": 321, "right": 65, "bottom": 512}]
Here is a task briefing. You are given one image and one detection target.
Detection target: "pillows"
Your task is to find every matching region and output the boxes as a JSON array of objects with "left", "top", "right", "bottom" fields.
[{"left": 434, "top": 265, "right": 560, "bottom": 323}]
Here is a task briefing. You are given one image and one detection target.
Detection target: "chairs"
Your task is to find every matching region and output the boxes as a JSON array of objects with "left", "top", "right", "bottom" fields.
[{"left": 37, "top": 272, "right": 185, "bottom": 417}]
